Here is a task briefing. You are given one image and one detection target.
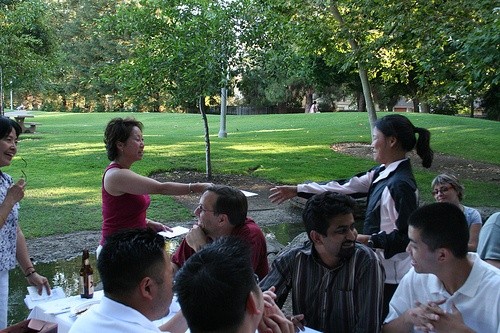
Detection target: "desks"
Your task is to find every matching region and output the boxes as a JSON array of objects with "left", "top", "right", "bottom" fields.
[{"left": 14, "top": 113, "right": 34, "bottom": 132}]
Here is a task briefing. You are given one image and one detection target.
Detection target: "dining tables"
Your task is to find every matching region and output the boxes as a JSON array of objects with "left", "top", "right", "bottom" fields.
[{"left": 26, "top": 291, "right": 320, "bottom": 333}]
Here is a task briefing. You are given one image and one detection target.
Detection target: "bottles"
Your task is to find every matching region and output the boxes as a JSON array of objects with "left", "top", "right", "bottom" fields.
[{"left": 80, "top": 250, "right": 94, "bottom": 299}]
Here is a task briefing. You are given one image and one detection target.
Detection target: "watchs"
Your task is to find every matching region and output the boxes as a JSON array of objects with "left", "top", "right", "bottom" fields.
[{"left": 366, "top": 235, "right": 373, "bottom": 247}]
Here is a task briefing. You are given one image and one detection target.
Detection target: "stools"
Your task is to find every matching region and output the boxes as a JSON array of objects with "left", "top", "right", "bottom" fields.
[{"left": 30, "top": 122, "right": 36, "bottom": 131}]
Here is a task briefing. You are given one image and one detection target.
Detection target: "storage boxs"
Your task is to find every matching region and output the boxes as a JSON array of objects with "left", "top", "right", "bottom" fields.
[{"left": 0, "top": 318, "right": 58, "bottom": 333}]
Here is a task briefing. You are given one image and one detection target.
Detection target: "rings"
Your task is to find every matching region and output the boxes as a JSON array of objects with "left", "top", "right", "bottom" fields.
[{"left": 290, "top": 316, "right": 295, "bottom": 320}]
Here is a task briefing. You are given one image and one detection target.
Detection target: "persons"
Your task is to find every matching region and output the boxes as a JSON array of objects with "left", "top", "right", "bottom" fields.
[
  {"left": 67, "top": 173, "right": 500, "bottom": 333},
  {"left": 310, "top": 101, "right": 319, "bottom": 114},
  {"left": 268, "top": 114, "right": 435, "bottom": 318},
  {"left": 0, "top": 116, "right": 50, "bottom": 331},
  {"left": 95, "top": 117, "right": 218, "bottom": 262}
]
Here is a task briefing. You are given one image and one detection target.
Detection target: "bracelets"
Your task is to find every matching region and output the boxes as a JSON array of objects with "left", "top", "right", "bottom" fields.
[
  {"left": 188, "top": 182, "right": 192, "bottom": 194},
  {"left": 25, "top": 267, "right": 36, "bottom": 277}
]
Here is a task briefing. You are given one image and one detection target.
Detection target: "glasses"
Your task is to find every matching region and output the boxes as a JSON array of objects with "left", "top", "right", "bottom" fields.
[
  {"left": 243, "top": 274, "right": 259, "bottom": 312},
  {"left": 432, "top": 186, "right": 454, "bottom": 197},
  {"left": 20, "top": 158, "right": 28, "bottom": 183},
  {"left": 197, "top": 204, "right": 214, "bottom": 213}
]
